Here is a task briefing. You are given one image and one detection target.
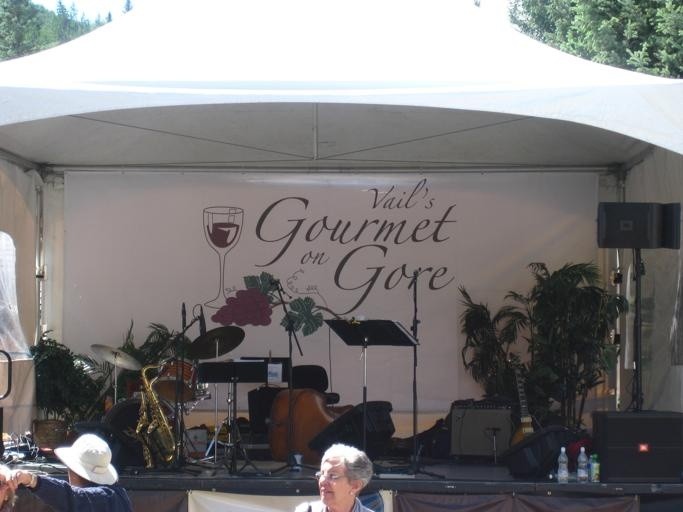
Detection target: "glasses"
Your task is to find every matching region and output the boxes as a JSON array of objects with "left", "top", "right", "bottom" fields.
[{"left": 315, "top": 472, "right": 348, "bottom": 483}]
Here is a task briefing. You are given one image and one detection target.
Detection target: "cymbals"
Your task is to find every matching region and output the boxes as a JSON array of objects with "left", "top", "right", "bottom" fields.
[
  {"left": 90, "top": 344, "right": 141, "bottom": 372},
  {"left": 187, "top": 324, "right": 244, "bottom": 359}
]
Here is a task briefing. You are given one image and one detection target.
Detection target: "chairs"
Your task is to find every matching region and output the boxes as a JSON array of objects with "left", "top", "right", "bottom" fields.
[{"left": 292, "top": 365, "right": 339, "bottom": 405}]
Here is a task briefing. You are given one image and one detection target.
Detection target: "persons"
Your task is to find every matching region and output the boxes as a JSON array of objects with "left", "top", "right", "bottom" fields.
[
  {"left": 293, "top": 442, "right": 375, "bottom": 512},
  {"left": 5, "top": 432, "right": 133, "bottom": 512},
  {"left": 0, "top": 464, "right": 18, "bottom": 511}
]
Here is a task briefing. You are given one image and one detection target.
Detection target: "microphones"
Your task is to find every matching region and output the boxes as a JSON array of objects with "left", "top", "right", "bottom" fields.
[
  {"left": 199, "top": 305, "right": 206, "bottom": 340},
  {"left": 267, "top": 275, "right": 279, "bottom": 291},
  {"left": 407, "top": 269, "right": 419, "bottom": 290}
]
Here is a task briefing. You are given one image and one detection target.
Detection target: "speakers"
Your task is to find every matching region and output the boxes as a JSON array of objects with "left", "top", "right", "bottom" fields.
[
  {"left": 450, "top": 402, "right": 514, "bottom": 458},
  {"left": 591, "top": 409, "right": 683, "bottom": 484},
  {"left": 596, "top": 200, "right": 681, "bottom": 251},
  {"left": 309, "top": 401, "right": 396, "bottom": 460}
]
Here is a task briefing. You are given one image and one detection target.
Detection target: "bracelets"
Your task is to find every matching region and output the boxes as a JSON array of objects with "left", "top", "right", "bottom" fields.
[{"left": 25, "top": 472, "right": 34, "bottom": 487}]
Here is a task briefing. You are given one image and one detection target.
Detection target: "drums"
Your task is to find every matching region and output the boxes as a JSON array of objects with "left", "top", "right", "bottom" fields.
[
  {"left": 152, "top": 358, "right": 199, "bottom": 402},
  {"left": 104, "top": 393, "right": 179, "bottom": 467}
]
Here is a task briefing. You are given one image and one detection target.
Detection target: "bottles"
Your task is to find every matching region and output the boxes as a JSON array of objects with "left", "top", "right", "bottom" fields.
[
  {"left": 576, "top": 446, "right": 589, "bottom": 484},
  {"left": 557, "top": 446, "right": 569, "bottom": 484},
  {"left": 590, "top": 453, "right": 601, "bottom": 483},
  {"left": 588, "top": 455, "right": 594, "bottom": 481}
]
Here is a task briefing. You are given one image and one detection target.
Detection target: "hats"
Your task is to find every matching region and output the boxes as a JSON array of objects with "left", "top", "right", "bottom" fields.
[{"left": 53, "top": 432, "right": 119, "bottom": 486}]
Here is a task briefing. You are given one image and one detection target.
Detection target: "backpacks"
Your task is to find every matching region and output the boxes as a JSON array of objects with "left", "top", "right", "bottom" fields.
[{"left": 502, "top": 425, "right": 586, "bottom": 479}]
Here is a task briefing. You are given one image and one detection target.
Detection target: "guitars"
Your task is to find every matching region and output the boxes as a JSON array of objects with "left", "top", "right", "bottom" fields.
[{"left": 507, "top": 352, "right": 543, "bottom": 448}]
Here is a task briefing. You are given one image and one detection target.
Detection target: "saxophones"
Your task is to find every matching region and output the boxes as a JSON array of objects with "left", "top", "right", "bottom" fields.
[{"left": 134, "top": 353, "right": 175, "bottom": 468}]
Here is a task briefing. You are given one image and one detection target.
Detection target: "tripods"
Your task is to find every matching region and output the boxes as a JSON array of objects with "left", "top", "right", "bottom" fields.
[
  {"left": 268, "top": 324, "right": 321, "bottom": 475},
  {"left": 390, "top": 284, "right": 445, "bottom": 479},
  {"left": 211, "top": 377, "right": 267, "bottom": 479},
  {"left": 205, "top": 381, "right": 247, "bottom": 459},
  {"left": 349, "top": 337, "right": 389, "bottom": 474}
]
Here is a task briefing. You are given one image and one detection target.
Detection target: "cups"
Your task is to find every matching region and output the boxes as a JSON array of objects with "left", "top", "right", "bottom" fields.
[{"left": 268, "top": 364, "right": 283, "bottom": 384}]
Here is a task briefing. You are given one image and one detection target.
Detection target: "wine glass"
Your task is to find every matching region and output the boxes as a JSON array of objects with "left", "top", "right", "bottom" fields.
[{"left": 203, "top": 206, "right": 244, "bottom": 308}]
